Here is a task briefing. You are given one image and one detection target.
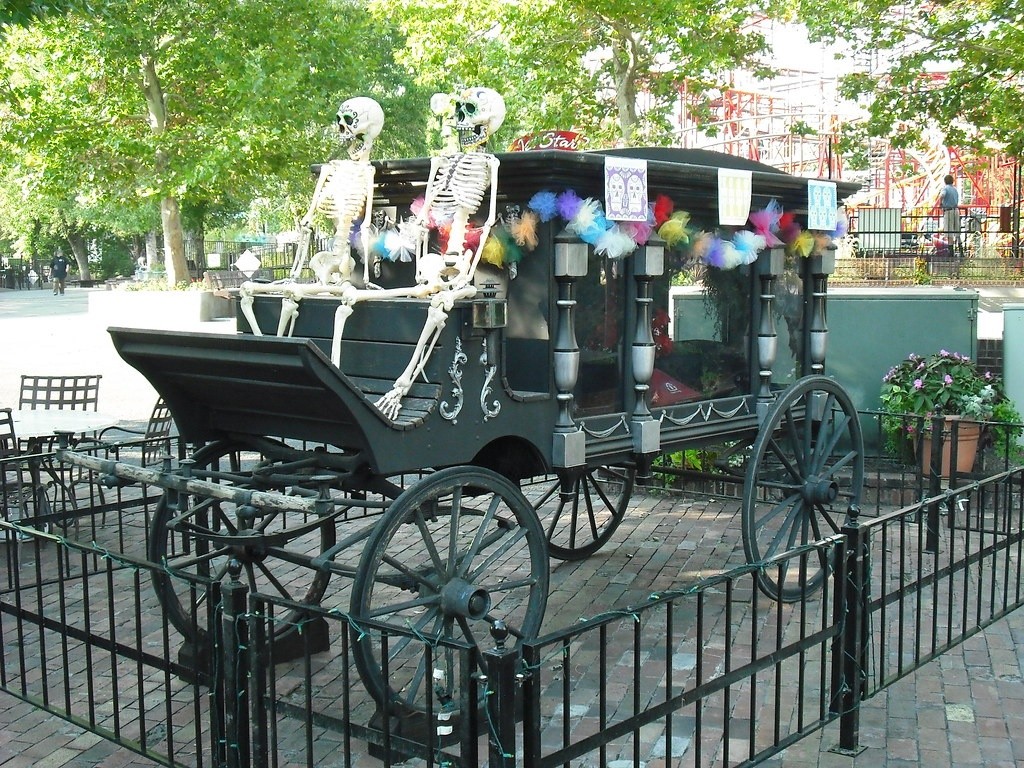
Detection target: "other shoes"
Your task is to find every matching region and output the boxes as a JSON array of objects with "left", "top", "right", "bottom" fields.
[
  {"left": 54, "top": 293, "right": 57, "bottom": 295},
  {"left": 61, "top": 293, "right": 64, "bottom": 296}
]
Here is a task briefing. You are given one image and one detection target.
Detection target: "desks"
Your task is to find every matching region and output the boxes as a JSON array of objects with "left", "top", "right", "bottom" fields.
[{"left": 0, "top": 410, "right": 119, "bottom": 537}]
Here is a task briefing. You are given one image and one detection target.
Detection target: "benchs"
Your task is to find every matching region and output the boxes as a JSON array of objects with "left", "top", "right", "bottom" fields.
[
  {"left": 234, "top": 275, "right": 509, "bottom": 411},
  {"left": 70, "top": 280, "right": 102, "bottom": 288}
]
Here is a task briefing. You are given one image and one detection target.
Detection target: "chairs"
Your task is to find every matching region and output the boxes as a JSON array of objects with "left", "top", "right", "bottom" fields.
[
  {"left": 18, "top": 374, "right": 106, "bottom": 529},
  {"left": 64, "top": 394, "right": 172, "bottom": 541},
  {"left": 0, "top": 406, "right": 52, "bottom": 570}
]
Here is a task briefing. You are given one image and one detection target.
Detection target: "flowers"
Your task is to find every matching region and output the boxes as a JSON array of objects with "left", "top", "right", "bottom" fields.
[{"left": 876, "top": 350, "right": 1024, "bottom": 462}]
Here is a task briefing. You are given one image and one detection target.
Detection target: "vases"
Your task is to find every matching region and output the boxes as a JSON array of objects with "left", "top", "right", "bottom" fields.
[{"left": 914, "top": 413, "right": 978, "bottom": 480}]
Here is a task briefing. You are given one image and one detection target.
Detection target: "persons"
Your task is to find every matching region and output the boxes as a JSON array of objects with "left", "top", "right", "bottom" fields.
[
  {"left": 47, "top": 248, "right": 70, "bottom": 296},
  {"left": 930, "top": 176, "right": 966, "bottom": 259}
]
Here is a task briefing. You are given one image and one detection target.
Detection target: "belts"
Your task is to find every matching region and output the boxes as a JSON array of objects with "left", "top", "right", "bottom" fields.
[{"left": 944, "top": 209, "right": 952, "bottom": 212}]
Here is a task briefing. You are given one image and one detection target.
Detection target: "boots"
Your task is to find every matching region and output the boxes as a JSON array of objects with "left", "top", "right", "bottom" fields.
[
  {"left": 958, "top": 243, "right": 964, "bottom": 257},
  {"left": 948, "top": 246, "right": 954, "bottom": 257}
]
